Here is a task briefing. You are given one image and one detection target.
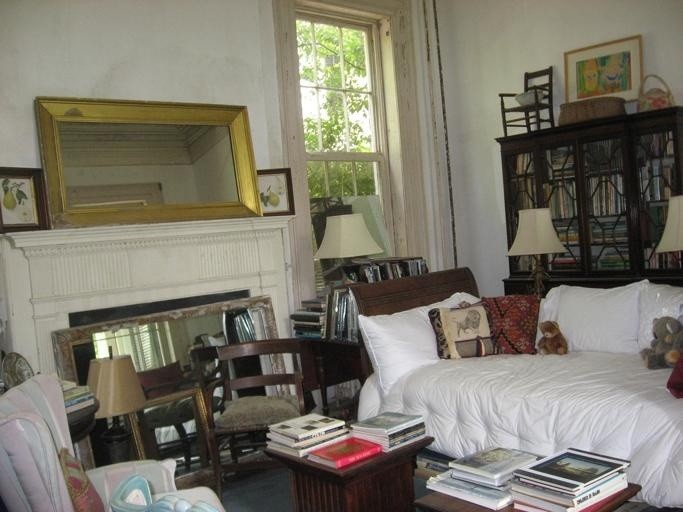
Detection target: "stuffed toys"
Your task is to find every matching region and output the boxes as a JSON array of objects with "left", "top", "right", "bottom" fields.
[
  {"left": 665, "top": 329, "right": 683, "bottom": 368},
  {"left": 640, "top": 317, "right": 680, "bottom": 370},
  {"left": 538, "top": 320, "right": 568, "bottom": 355}
]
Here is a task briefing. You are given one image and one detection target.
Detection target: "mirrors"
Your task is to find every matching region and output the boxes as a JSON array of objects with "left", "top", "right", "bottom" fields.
[
  {"left": 127, "top": 387, "right": 226, "bottom": 490},
  {"left": 49, "top": 296, "right": 291, "bottom": 479},
  {"left": 34, "top": 96, "right": 264, "bottom": 230}
]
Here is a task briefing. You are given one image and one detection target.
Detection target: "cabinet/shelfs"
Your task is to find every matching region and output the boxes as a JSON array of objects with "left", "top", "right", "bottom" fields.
[
  {"left": 298, "top": 332, "right": 362, "bottom": 418},
  {"left": 495, "top": 107, "right": 683, "bottom": 276}
]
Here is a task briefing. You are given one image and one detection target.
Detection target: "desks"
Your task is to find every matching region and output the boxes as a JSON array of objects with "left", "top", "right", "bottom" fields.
[
  {"left": 415, "top": 437, "right": 641, "bottom": 512},
  {"left": 264, "top": 436, "right": 435, "bottom": 511}
]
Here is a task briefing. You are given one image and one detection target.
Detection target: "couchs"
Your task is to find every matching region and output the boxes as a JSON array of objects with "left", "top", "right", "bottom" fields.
[{"left": 357, "top": 283, "right": 682, "bottom": 512}]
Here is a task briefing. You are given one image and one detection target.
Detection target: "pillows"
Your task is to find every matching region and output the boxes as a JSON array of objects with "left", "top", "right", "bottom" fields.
[
  {"left": 535, "top": 279, "right": 649, "bottom": 354},
  {"left": 215, "top": 396, "right": 302, "bottom": 429},
  {"left": 483, "top": 295, "right": 539, "bottom": 354},
  {"left": 428, "top": 302, "right": 494, "bottom": 359},
  {"left": 358, "top": 292, "right": 483, "bottom": 400},
  {"left": 638, "top": 282, "right": 683, "bottom": 354}
]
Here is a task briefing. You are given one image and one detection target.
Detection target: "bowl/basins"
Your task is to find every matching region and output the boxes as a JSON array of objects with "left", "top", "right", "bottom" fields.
[{"left": 513, "top": 90, "right": 545, "bottom": 107}]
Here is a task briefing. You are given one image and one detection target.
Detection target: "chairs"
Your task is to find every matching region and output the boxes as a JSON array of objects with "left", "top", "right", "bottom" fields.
[
  {"left": 498, "top": 66, "right": 554, "bottom": 137},
  {"left": 204, "top": 339, "right": 308, "bottom": 502},
  {"left": 0, "top": 372, "right": 227, "bottom": 512}
]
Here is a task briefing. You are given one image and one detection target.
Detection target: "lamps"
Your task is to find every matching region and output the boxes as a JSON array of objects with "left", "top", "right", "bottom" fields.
[
  {"left": 93, "top": 355, "right": 147, "bottom": 462},
  {"left": 86, "top": 355, "right": 147, "bottom": 441},
  {"left": 655, "top": 196, "right": 683, "bottom": 254},
  {"left": 505, "top": 209, "right": 570, "bottom": 297},
  {"left": 311, "top": 213, "right": 383, "bottom": 278}
]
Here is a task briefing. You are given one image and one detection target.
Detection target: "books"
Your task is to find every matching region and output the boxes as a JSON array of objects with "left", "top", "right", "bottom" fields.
[
  {"left": 290, "top": 255, "right": 429, "bottom": 342},
  {"left": 264, "top": 409, "right": 427, "bottom": 470},
  {"left": 61, "top": 380, "right": 95, "bottom": 414},
  {"left": 426, "top": 447, "right": 633, "bottom": 511},
  {"left": 509, "top": 132, "right": 683, "bottom": 272}
]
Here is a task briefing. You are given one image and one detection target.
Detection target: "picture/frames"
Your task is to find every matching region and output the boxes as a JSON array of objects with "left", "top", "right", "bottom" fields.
[
  {"left": 0, "top": 167, "right": 50, "bottom": 234},
  {"left": 256, "top": 168, "right": 295, "bottom": 216},
  {"left": 564, "top": 34, "right": 643, "bottom": 103}
]
